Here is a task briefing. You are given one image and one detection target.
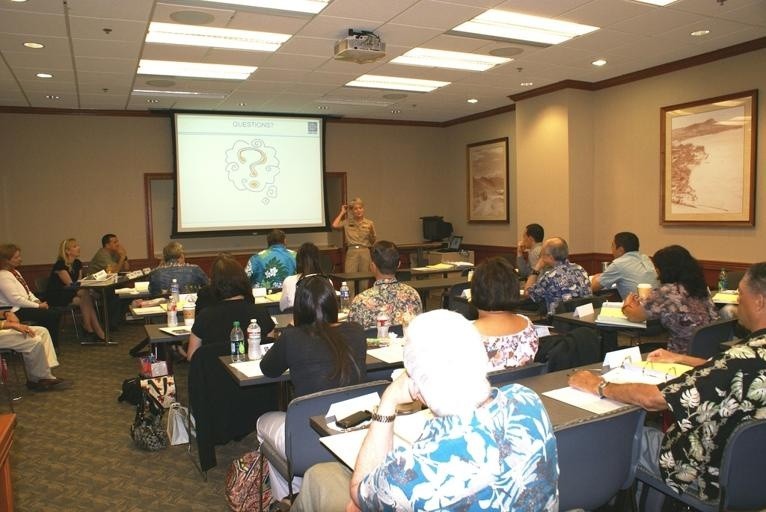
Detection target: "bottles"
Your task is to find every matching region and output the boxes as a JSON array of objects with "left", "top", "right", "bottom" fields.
[
  {"left": 718, "top": 267, "right": 728, "bottom": 293},
  {"left": 165, "top": 276, "right": 179, "bottom": 326},
  {"left": 246, "top": 318, "right": 262, "bottom": 359},
  {"left": 229, "top": 320, "right": 246, "bottom": 361},
  {"left": 339, "top": 281, "right": 350, "bottom": 314},
  {"left": 377, "top": 308, "right": 389, "bottom": 337}
]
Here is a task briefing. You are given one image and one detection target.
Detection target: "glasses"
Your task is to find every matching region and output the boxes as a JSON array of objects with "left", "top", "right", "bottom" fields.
[{"left": 296, "top": 275, "right": 329, "bottom": 288}]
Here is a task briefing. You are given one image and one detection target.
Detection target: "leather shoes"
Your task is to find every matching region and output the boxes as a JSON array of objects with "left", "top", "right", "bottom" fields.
[
  {"left": 26, "top": 379, "right": 49, "bottom": 391},
  {"left": 37, "top": 376, "right": 72, "bottom": 390}
]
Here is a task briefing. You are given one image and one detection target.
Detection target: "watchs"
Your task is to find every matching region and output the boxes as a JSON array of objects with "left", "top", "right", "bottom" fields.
[
  {"left": 598, "top": 381, "right": 609, "bottom": 397},
  {"left": 371, "top": 406, "right": 396, "bottom": 423}
]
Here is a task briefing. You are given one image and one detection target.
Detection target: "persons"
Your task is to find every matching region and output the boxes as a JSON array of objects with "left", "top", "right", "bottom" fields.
[
  {"left": 623, "top": 245, "right": 720, "bottom": 355},
  {"left": 333, "top": 198, "right": 377, "bottom": 299},
  {"left": 45, "top": 238, "right": 112, "bottom": 342},
  {"left": 243, "top": 229, "right": 298, "bottom": 288},
  {"left": 523, "top": 237, "right": 591, "bottom": 326},
  {"left": 592, "top": 231, "right": 659, "bottom": 302},
  {"left": 469, "top": 257, "right": 539, "bottom": 373},
  {"left": 257, "top": 277, "right": 367, "bottom": 512},
  {"left": 289, "top": 308, "right": 560, "bottom": 512},
  {"left": 0, "top": 311, "right": 65, "bottom": 390},
  {"left": 280, "top": 243, "right": 335, "bottom": 313},
  {"left": 148, "top": 242, "right": 210, "bottom": 299},
  {"left": 349, "top": 241, "right": 423, "bottom": 328},
  {"left": 0, "top": 243, "right": 61, "bottom": 347},
  {"left": 187, "top": 252, "right": 276, "bottom": 363},
  {"left": 87, "top": 234, "right": 130, "bottom": 329},
  {"left": 517, "top": 223, "right": 553, "bottom": 278},
  {"left": 570, "top": 262, "right": 766, "bottom": 511}
]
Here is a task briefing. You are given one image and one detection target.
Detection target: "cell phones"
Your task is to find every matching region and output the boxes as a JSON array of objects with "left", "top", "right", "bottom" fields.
[{"left": 336, "top": 411, "right": 371, "bottom": 429}]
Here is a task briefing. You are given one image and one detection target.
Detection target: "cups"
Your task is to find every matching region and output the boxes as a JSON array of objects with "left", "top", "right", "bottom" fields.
[
  {"left": 182, "top": 302, "right": 196, "bottom": 326},
  {"left": 636, "top": 282, "right": 652, "bottom": 299}
]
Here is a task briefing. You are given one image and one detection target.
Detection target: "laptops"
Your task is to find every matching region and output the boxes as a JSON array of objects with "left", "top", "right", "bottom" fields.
[{"left": 437, "top": 236, "right": 463, "bottom": 252}]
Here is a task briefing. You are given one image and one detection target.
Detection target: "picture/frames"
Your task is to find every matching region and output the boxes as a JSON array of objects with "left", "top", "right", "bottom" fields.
[
  {"left": 459, "top": 136, "right": 512, "bottom": 224},
  {"left": 652, "top": 87, "right": 758, "bottom": 230}
]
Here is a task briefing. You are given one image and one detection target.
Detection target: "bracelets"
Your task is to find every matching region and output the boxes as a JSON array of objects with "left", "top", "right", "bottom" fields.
[
  {"left": 1, "top": 320, "right": 7, "bottom": 328},
  {"left": 125, "top": 256, "right": 128, "bottom": 261},
  {"left": 621, "top": 305, "right": 632, "bottom": 314},
  {"left": 530, "top": 269, "right": 540, "bottom": 276},
  {"left": 3, "top": 311, "right": 10, "bottom": 316}
]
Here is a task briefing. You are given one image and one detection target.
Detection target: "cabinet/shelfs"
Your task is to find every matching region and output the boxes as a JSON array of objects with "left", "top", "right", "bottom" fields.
[{"left": 409, "top": 251, "right": 475, "bottom": 298}]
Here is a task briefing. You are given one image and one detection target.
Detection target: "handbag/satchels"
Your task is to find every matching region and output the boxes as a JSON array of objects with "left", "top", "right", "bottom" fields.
[
  {"left": 117, "top": 353, "right": 197, "bottom": 452},
  {"left": 224, "top": 444, "right": 274, "bottom": 512}
]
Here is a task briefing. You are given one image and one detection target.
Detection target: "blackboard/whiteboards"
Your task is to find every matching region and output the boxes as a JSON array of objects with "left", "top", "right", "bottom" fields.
[{"left": 143, "top": 172, "right": 348, "bottom": 260}]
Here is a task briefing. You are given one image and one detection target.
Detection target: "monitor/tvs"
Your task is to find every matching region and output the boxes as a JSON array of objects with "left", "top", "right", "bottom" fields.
[{"left": 423, "top": 218, "right": 453, "bottom": 241}]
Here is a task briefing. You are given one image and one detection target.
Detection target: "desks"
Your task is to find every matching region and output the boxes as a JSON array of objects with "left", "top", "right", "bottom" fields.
[{"left": 113, "top": 257, "right": 765, "bottom": 512}]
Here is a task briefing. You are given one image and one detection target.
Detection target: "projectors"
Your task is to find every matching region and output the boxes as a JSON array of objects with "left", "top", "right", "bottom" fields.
[{"left": 334, "top": 40, "right": 386, "bottom": 64}]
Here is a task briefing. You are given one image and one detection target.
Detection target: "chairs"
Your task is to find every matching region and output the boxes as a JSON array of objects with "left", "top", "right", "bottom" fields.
[{"left": 0, "top": 267, "right": 120, "bottom": 419}]
